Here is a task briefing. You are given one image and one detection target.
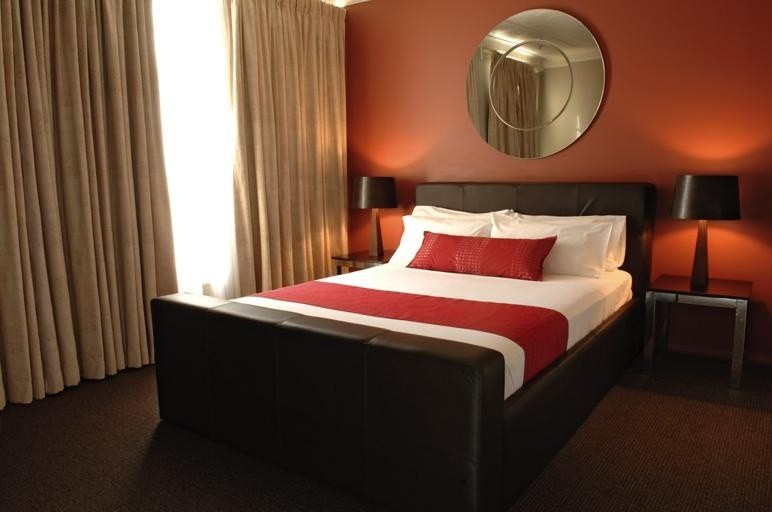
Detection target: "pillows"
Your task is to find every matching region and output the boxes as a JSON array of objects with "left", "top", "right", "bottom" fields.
[{"left": 386, "top": 204, "right": 631, "bottom": 283}]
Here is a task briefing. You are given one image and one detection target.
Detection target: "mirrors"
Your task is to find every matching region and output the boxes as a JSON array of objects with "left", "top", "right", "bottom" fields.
[{"left": 464, "top": 8, "right": 606, "bottom": 159}]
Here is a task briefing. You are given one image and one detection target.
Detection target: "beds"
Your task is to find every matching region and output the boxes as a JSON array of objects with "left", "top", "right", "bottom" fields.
[{"left": 149, "top": 177, "right": 659, "bottom": 510}]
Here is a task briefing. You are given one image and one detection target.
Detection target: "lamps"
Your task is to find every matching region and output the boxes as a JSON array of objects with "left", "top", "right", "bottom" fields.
[
  {"left": 666, "top": 171, "right": 742, "bottom": 291},
  {"left": 348, "top": 176, "right": 397, "bottom": 258}
]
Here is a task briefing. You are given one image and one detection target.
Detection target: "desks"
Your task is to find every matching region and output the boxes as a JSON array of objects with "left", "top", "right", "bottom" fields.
[
  {"left": 640, "top": 275, "right": 753, "bottom": 393},
  {"left": 329, "top": 250, "right": 392, "bottom": 276}
]
